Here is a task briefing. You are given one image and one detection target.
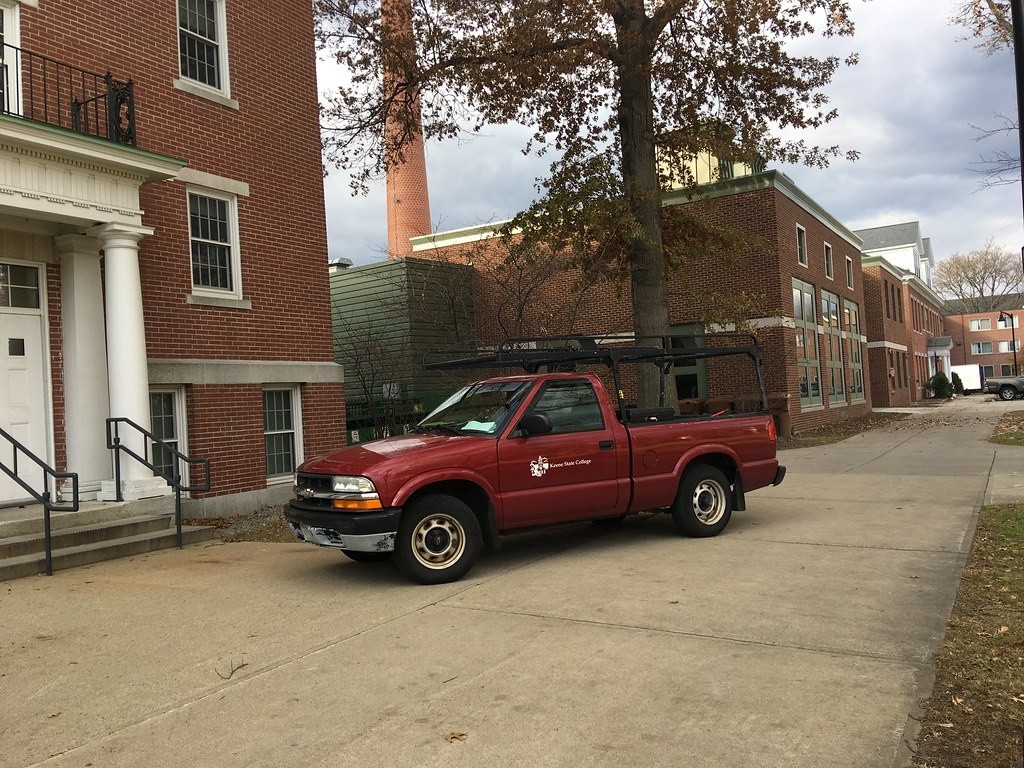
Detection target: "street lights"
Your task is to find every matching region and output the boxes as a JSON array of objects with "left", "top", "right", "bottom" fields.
[{"left": 998, "top": 309, "right": 1018, "bottom": 377}]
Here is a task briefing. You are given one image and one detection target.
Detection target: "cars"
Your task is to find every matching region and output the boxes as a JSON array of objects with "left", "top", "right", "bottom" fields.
[{"left": 983, "top": 379, "right": 989, "bottom": 394}]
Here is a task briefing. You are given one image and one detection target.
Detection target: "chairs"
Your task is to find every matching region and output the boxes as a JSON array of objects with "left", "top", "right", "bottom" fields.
[{"left": 566, "top": 394, "right": 604, "bottom": 432}]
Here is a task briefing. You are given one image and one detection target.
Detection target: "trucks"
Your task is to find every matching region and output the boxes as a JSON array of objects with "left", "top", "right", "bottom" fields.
[{"left": 951, "top": 364, "right": 983, "bottom": 395}]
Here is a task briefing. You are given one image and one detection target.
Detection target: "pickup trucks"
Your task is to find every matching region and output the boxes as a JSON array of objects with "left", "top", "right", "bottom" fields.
[
  {"left": 282, "top": 328, "right": 788, "bottom": 584},
  {"left": 984, "top": 372, "right": 1024, "bottom": 402}
]
